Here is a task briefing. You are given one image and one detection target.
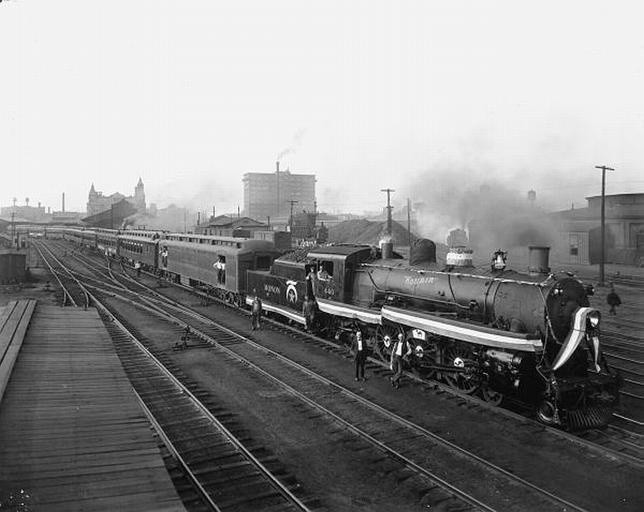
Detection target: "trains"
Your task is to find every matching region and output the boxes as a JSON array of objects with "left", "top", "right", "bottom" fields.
[{"left": 4, "top": 223, "right": 627, "bottom": 435}]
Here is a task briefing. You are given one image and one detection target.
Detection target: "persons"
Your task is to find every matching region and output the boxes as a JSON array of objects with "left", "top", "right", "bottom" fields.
[
  {"left": 389, "top": 333, "right": 413, "bottom": 389},
  {"left": 220, "top": 258, "right": 225, "bottom": 284},
  {"left": 350, "top": 330, "right": 368, "bottom": 382},
  {"left": 317, "top": 264, "right": 332, "bottom": 282},
  {"left": 250, "top": 295, "right": 263, "bottom": 330},
  {"left": 607, "top": 288, "right": 622, "bottom": 316},
  {"left": 306, "top": 266, "right": 317, "bottom": 297},
  {"left": 134, "top": 260, "right": 142, "bottom": 277},
  {"left": 156, "top": 247, "right": 169, "bottom": 266},
  {"left": 212, "top": 257, "right": 224, "bottom": 282},
  {"left": 302, "top": 292, "right": 315, "bottom": 334}
]
